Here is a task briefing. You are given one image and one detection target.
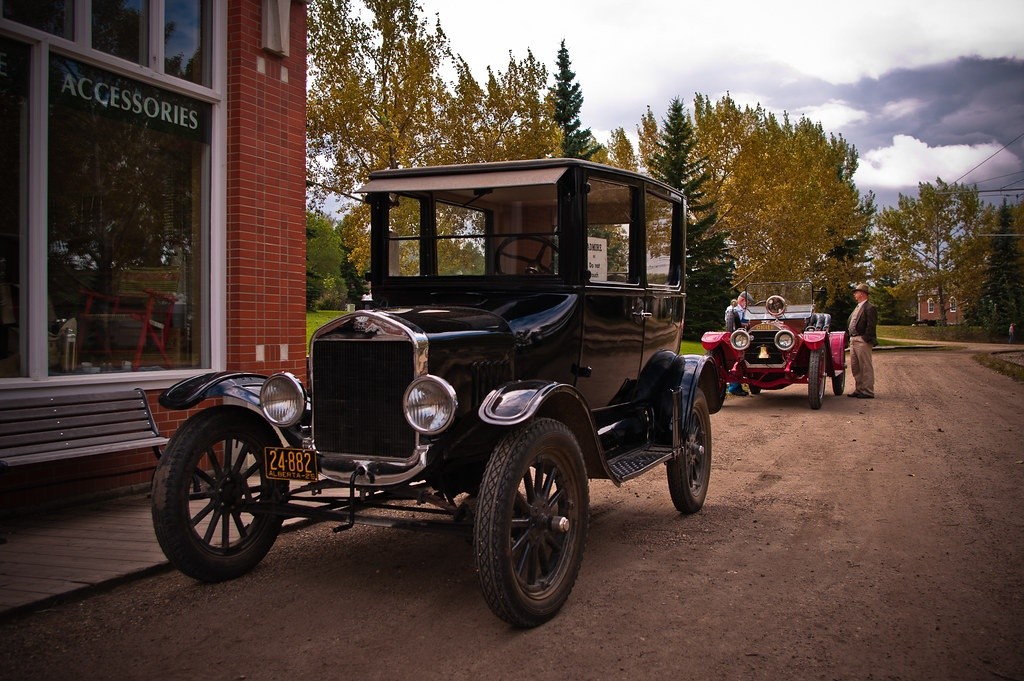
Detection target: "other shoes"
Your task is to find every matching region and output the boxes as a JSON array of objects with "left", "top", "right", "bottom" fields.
[
  {"left": 847, "top": 392, "right": 858, "bottom": 397},
  {"left": 856, "top": 393, "right": 873, "bottom": 398},
  {"left": 729, "top": 388, "right": 748, "bottom": 396}
]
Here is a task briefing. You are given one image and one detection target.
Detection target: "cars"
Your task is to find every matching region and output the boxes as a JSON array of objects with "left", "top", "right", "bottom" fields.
[
  {"left": 701, "top": 281, "right": 847, "bottom": 410},
  {"left": 152, "top": 158, "right": 723, "bottom": 627}
]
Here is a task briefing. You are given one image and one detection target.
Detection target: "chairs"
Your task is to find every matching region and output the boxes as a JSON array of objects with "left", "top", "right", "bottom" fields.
[{"left": 76, "top": 265, "right": 182, "bottom": 373}]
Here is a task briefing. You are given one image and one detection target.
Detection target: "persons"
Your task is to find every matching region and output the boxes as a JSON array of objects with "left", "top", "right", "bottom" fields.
[
  {"left": 724, "top": 291, "right": 754, "bottom": 397},
  {"left": 847, "top": 284, "right": 879, "bottom": 399},
  {"left": 1007, "top": 323, "right": 1017, "bottom": 344}
]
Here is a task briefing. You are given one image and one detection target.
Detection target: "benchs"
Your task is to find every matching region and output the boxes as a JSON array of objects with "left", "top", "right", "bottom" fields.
[{"left": 0, "top": 387, "right": 201, "bottom": 544}]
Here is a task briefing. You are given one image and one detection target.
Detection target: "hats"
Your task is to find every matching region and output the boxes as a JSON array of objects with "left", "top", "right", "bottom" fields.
[
  {"left": 740, "top": 291, "right": 754, "bottom": 302},
  {"left": 851, "top": 283, "right": 871, "bottom": 294}
]
